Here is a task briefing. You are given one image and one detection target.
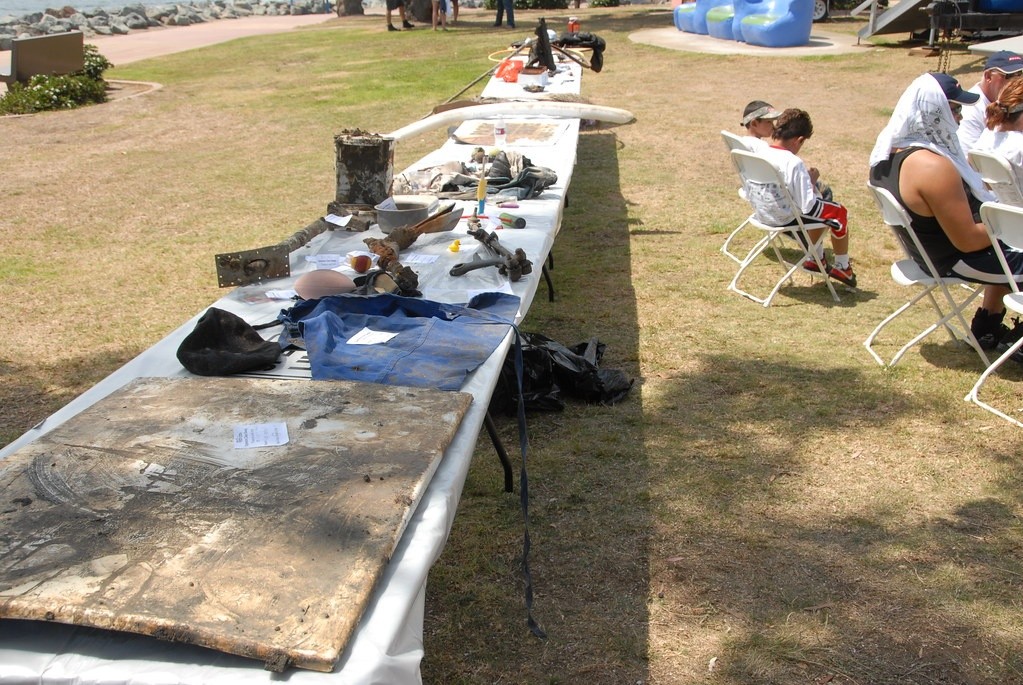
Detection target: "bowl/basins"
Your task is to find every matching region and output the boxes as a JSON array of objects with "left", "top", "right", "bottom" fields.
[
  {"left": 414, "top": 204, "right": 464, "bottom": 232},
  {"left": 375, "top": 202, "right": 429, "bottom": 234}
]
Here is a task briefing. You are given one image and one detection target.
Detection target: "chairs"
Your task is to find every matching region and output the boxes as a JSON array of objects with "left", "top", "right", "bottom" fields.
[
  {"left": 862, "top": 180, "right": 995, "bottom": 374},
  {"left": 728, "top": 148, "right": 841, "bottom": 307},
  {"left": 966, "top": 149, "right": 1023, "bottom": 208},
  {"left": 721, "top": 129, "right": 808, "bottom": 263},
  {"left": 965, "top": 201, "right": 1023, "bottom": 427},
  {"left": 674, "top": 0, "right": 815, "bottom": 46}
]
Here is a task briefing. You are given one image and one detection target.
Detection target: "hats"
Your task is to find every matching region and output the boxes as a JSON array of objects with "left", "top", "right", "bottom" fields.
[
  {"left": 928, "top": 72, "right": 981, "bottom": 106},
  {"left": 985, "top": 49, "right": 1023, "bottom": 73},
  {"left": 739, "top": 106, "right": 783, "bottom": 124}
]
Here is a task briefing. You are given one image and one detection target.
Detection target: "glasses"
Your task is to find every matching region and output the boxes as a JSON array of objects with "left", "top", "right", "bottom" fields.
[
  {"left": 950, "top": 105, "right": 962, "bottom": 113},
  {"left": 996, "top": 71, "right": 1022, "bottom": 79}
]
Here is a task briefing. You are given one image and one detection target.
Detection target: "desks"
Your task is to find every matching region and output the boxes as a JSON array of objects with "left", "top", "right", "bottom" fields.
[{"left": 0, "top": 56, "right": 581, "bottom": 685}]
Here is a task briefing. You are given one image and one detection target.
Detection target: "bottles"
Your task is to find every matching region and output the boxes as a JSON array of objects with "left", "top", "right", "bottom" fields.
[
  {"left": 493, "top": 114, "right": 507, "bottom": 151},
  {"left": 568, "top": 17, "right": 579, "bottom": 34}
]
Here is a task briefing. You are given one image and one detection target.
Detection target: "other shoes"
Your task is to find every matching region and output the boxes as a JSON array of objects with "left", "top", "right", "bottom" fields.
[{"left": 387, "top": 20, "right": 415, "bottom": 31}]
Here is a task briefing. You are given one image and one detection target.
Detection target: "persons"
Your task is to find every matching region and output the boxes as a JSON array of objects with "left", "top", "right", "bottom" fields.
[
  {"left": 494, "top": 0, "right": 515, "bottom": 28},
  {"left": 740, "top": 100, "right": 857, "bottom": 287},
  {"left": 869, "top": 50, "right": 1023, "bottom": 363},
  {"left": 386, "top": 0, "right": 416, "bottom": 31},
  {"left": 432, "top": 0, "right": 459, "bottom": 32}
]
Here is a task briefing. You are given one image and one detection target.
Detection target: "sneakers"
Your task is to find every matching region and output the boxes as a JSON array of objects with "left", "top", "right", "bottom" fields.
[
  {"left": 970, "top": 307, "right": 1023, "bottom": 362},
  {"left": 803, "top": 253, "right": 859, "bottom": 288}
]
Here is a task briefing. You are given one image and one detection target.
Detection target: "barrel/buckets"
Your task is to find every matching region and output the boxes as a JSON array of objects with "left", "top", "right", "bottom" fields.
[{"left": 335, "top": 133, "right": 395, "bottom": 209}]
